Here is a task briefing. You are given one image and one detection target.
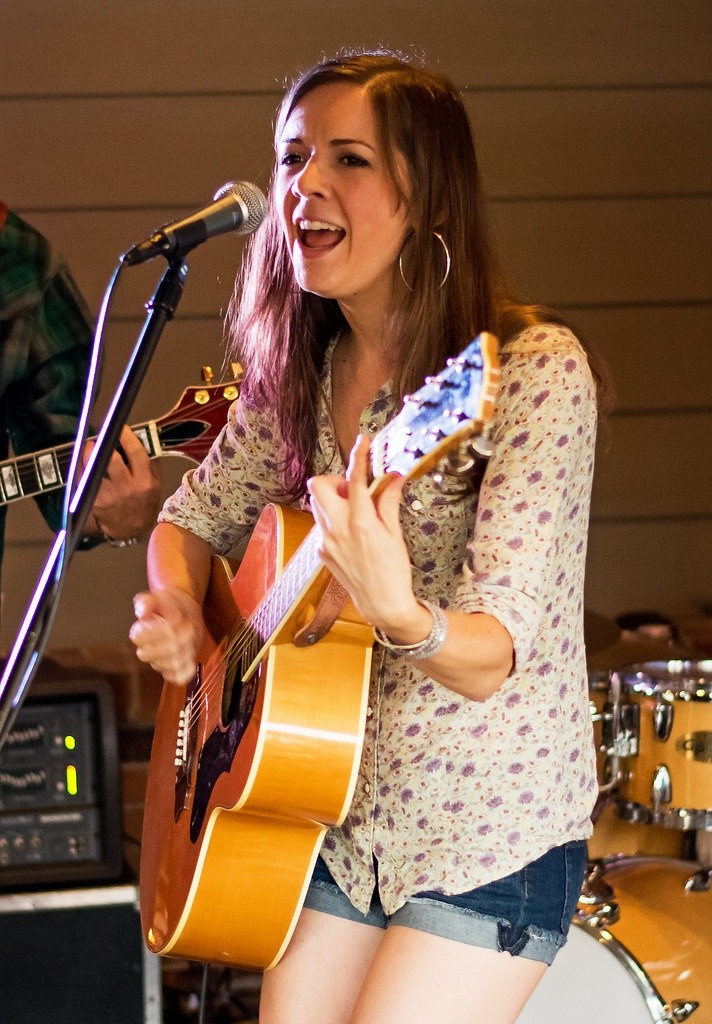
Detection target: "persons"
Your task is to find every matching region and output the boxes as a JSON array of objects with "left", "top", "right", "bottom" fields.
[
  {"left": 128, "top": 52, "right": 599, "bottom": 1024},
  {"left": 0, "top": 200, "right": 159, "bottom": 576}
]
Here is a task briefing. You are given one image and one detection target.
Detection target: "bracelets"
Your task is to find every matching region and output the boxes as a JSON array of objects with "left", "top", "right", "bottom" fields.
[
  {"left": 96, "top": 520, "right": 141, "bottom": 548},
  {"left": 371, "top": 600, "right": 449, "bottom": 659}
]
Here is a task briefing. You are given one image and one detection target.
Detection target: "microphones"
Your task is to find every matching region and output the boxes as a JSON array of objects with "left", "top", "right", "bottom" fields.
[{"left": 119, "top": 181, "right": 269, "bottom": 267}]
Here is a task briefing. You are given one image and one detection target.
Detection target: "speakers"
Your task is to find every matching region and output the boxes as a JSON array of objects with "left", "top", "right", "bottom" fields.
[{"left": 0, "top": 887, "right": 161, "bottom": 1024}]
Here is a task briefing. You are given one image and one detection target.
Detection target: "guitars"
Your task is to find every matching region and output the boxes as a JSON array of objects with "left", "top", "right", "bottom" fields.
[
  {"left": 0, "top": 362, "right": 241, "bottom": 503},
  {"left": 142, "top": 330, "right": 502, "bottom": 969}
]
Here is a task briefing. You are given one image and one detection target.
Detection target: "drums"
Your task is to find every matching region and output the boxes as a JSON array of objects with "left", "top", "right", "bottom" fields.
[
  {"left": 587, "top": 668, "right": 612, "bottom": 825},
  {"left": 512, "top": 857, "right": 711, "bottom": 1024},
  {"left": 609, "top": 657, "right": 711, "bottom": 828}
]
